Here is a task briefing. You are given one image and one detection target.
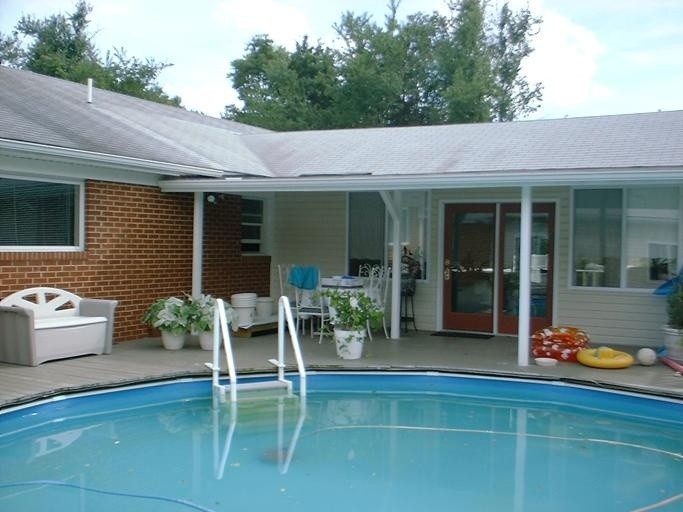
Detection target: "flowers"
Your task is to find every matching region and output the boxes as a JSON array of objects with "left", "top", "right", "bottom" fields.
[{"left": 141, "top": 291, "right": 236, "bottom": 338}]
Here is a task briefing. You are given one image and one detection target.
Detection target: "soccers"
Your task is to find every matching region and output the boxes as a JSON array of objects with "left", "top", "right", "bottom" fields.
[{"left": 637, "top": 348, "right": 656, "bottom": 366}]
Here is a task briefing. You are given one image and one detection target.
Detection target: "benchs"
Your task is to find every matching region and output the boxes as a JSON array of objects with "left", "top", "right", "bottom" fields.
[{"left": 0, "top": 286, "right": 118, "bottom": 369}]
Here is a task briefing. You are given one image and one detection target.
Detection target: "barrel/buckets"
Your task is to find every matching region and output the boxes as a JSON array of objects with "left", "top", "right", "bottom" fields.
[{"left": 229, "top": 291, "right": 275, "bottom": 324}]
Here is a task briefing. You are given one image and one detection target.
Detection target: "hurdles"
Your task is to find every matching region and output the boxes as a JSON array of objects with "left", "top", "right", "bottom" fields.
[{"left": 576, "top": 347, "right": 634, "bottom": 368}]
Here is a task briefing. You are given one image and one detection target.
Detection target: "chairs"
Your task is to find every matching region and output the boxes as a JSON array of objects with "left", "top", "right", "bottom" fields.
[
  {"left": 532, "top": 325, "right": 588, "bottom": 363},
  {"left": 277, "top": 263, "right": 391, "bottom": 344}
]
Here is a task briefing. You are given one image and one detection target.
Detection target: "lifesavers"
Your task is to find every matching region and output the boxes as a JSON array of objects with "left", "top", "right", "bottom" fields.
[{"left": 531, "top": 326, "right": 590, "bottom": 362}]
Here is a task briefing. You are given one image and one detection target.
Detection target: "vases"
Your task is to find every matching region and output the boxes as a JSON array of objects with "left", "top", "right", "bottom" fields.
[{"left": 159, "top": 328, "right": 221, "bottom": 352}]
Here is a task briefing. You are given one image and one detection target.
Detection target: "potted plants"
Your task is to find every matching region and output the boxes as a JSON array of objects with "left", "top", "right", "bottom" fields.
[
  {"left": 311, "top": 288, "right": 383, "bottom": 360},
  {"left": 660, "top": 282, "right": 682, "bottom": 360}
]
